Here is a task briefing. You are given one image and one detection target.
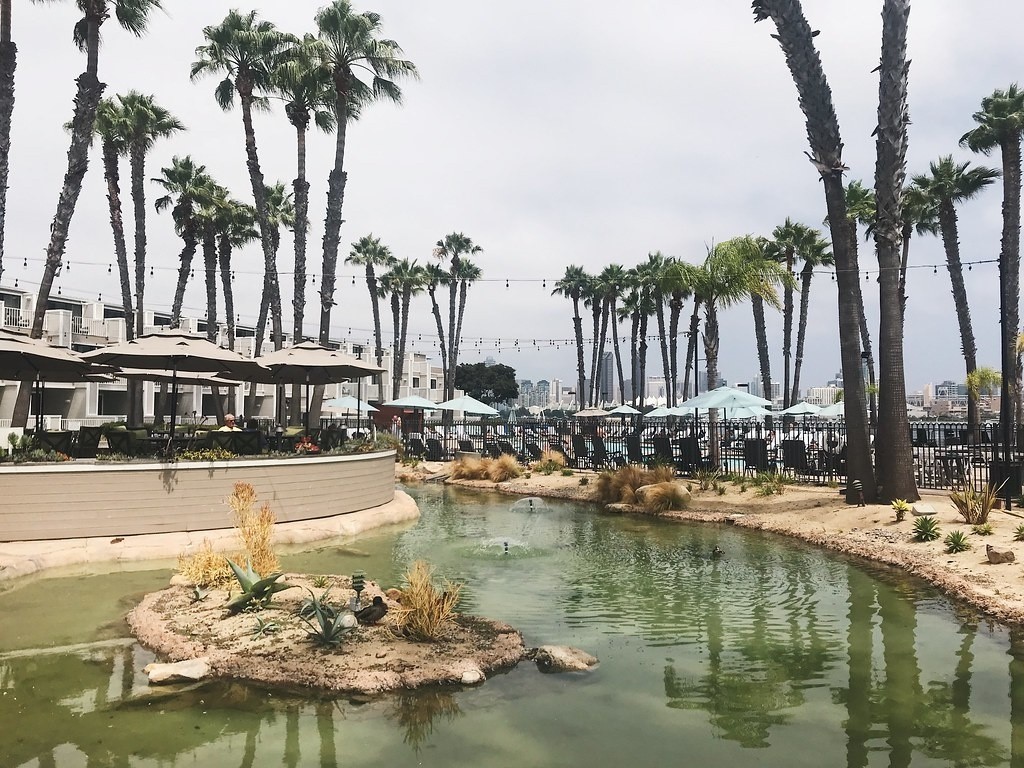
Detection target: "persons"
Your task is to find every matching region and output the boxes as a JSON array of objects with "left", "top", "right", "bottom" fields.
[
  {"left": 218, "top": 413, "right": 242, "bottom": 431},
  {"left": 763, "top": 430, "right": 775, "bottom": 443},
  {"left": 243, "top": 419, "right": 267, "bottom": 449}
]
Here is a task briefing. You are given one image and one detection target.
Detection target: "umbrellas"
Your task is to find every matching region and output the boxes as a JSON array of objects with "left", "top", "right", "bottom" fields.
[
  {"left": 381, "top": 394, "right": 500, "bottom": 433},
  {"left": 0, "top": 327, "right": 391, "bottom": 439},
  {"left": 678, "top": 384, "right": 772, "bottom": 475},
  {"left": 572, "top": 400, "right": 918, "bottom": 433}
]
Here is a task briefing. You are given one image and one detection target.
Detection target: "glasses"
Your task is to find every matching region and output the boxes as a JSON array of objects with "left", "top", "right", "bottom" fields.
[{"left": 228, "top": 420, "right": 235, "bottom": 422}]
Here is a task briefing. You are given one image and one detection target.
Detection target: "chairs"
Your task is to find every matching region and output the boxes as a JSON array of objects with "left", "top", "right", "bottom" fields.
[
  {"left": 25, "top": 426, "right": 347, "bottom": 457},
  {"left": 409, "top": 435, "right": 847, "bottom": 486},
  {"left": 909, "top": 427, "right": 1023, "bottom": 511}
]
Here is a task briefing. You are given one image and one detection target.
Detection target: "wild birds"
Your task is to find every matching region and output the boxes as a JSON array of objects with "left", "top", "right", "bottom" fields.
[{"left": 331, "top": 597, "right": 389, "bottom": 635}]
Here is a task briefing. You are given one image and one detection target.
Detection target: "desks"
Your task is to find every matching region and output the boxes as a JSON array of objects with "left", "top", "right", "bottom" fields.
[
  {"left": 38, "top": 429, "right": 73, "bottom": 455},
  {"left": 137, "top": 437, "right": 206, "bottom": 449},
  {"left": 265, "top": 435, "right": 301, "bottom": 439},
  {"left": 935, "top": 454, "right": 983, "bottom": 488}
]
